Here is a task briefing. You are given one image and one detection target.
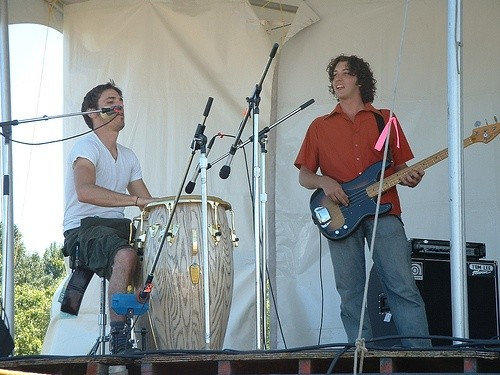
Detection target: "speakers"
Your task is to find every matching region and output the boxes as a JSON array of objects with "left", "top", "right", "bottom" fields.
[
  {"left": 0, "top": 315, "right": 15, "bottom": 357},
  {"left": 367, "top": 255, "right": 500, "bottom": 349}
]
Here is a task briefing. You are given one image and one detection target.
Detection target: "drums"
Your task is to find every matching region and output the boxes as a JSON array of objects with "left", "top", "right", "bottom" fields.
[{"left": 134, "top": 195, "right": 234, "bottom": 350}]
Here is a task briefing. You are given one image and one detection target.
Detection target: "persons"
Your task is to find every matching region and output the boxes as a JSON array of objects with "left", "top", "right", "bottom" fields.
[
  {"left": 293, "top": 53, "right": 432, "bottom": 350},
  {"left": 61, "top": 79, "right": 160, "bottom": 358}
]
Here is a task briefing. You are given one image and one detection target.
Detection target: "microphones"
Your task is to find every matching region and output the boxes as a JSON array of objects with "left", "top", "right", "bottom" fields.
[
  {"left": 185, "top": 136, "right": 215, "bottom": 194},
  {"left": 100, "top": 106, "right": 122, "bottom": 120},
  {"left": 219, "top": 143, "right": 237, "bottom": 179}
]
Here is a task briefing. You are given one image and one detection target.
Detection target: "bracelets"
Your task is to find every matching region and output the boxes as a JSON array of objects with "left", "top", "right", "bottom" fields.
[{"left": 135, "top": 196, "right": 139, "bottom": 207}]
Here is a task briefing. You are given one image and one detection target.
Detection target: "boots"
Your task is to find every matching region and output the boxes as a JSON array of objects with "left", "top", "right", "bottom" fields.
[{"left": 106, "top": 322, "right": 143, "bottom": 355}]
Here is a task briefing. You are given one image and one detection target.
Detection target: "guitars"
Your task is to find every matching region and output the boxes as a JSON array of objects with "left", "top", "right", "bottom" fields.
[{"left": 309, "top": 122, "right": 500, "bottom": 241}]
